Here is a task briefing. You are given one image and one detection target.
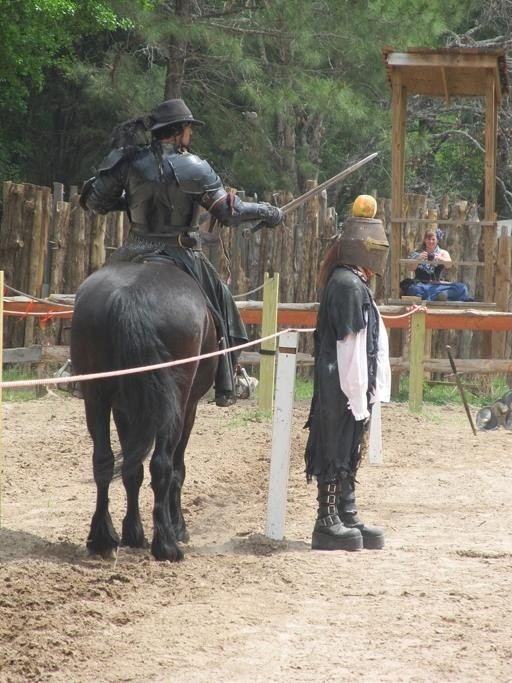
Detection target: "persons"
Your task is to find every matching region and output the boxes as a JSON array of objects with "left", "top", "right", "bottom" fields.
[
  {"left": 303, "top": 219, "right": 391, "bottom": 550},
  {"left": 80, "top": 98, "right": 283, "bottom": 407},
  {"left": 399, "top": 229, "right": 474, "bottom": 301}
]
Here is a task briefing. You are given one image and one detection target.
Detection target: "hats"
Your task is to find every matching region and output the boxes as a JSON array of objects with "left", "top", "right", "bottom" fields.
[
  {"left": 261, "top": 202, "right": 284, "bottom": 227},
  {"left": 149, "top": 98, "right": 206, "bottom": 132}
]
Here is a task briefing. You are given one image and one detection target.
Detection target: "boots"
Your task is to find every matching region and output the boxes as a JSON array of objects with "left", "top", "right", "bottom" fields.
[{"left": 312, "top": 471, "right": 385, "bottom": 551}]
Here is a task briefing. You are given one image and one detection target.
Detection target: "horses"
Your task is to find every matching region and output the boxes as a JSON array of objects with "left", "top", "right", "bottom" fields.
[{"left": 68, "top": 252, "right": 222, "bottom": 564}]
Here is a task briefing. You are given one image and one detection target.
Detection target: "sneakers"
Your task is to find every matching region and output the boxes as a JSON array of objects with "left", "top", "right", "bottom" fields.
[
  {"left": 217, "top": 376, "right": 259, "bottom": 407},
  {"left": 54, "top": 361, "right": 84, "bottom": 399}
]
